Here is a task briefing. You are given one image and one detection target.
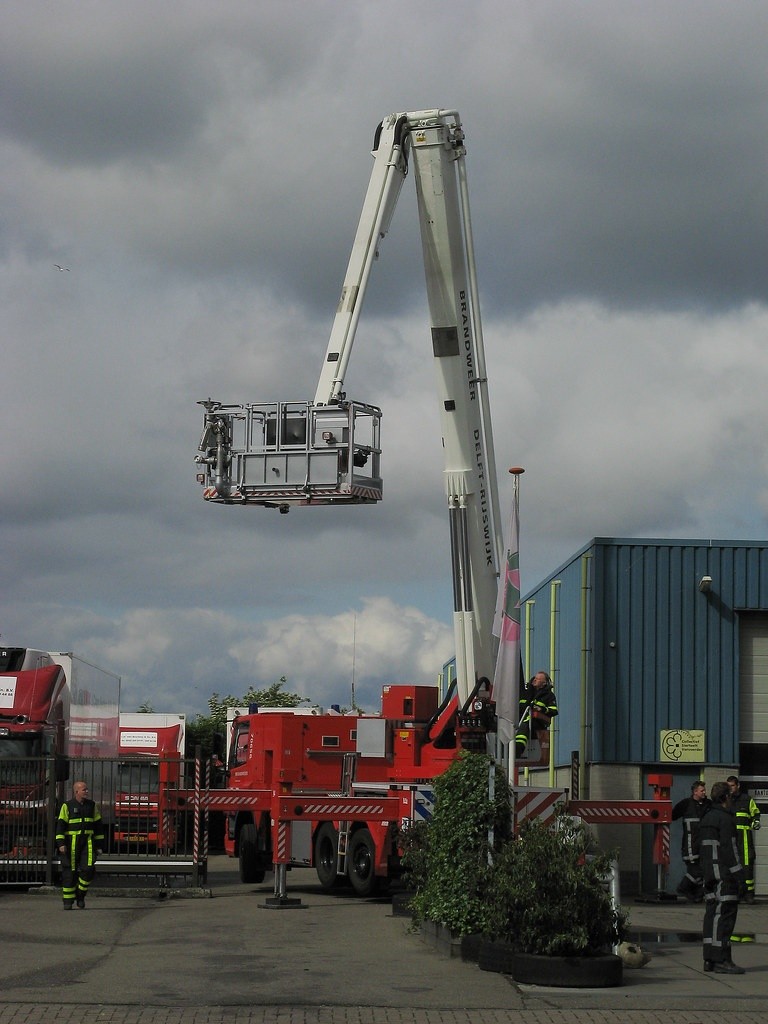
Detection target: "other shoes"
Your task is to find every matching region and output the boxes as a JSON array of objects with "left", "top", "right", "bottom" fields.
[
  {"left": 75, "top": 895, "right": 85, "bottom": 907},
  {"left": 64, "top": 903, "right": 72, "bottom": 910},
  {"left": 677, "top": 896, "right": 688, "bottom": 904},
  {"left": 745, "top": 894, "right": 754, "bottom": 904},
  {"left": 694, "top": 897, "right": 702, "bottom": 903}
]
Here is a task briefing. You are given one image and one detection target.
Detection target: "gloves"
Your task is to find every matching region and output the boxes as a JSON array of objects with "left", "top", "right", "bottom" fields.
[
  {"left": 736, "top": 880, "right": 748, "bottom": 899},
  {"left": 753, "top": 819, "right": 761, "bottom": 830}
]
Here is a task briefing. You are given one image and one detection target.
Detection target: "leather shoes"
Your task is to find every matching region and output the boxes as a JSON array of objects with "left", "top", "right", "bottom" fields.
[
  {"left": 704, "top": 961, "right": 715, "bottom": 971},
  {"left": 715, "top": 960, "right": 745, "bottom": 974}
]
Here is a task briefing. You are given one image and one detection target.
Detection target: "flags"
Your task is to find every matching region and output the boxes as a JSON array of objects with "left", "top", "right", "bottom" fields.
[{"left": 490, "top": 489, "right": 521, "bottom": 744}]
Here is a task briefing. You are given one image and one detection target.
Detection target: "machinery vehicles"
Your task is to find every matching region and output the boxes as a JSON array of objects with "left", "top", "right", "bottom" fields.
[{"left": 153, "top": 106, "right": 675, "bottom": 903}]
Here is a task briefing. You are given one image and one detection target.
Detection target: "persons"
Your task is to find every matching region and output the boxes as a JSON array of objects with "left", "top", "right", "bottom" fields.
[
  {"left": 55, "top": 782, "right": 104, "bottom": 910},
  {"left": 727, "top": 776, "right": 761, "bottom": 904},
  {"left": 671, "top": 781, "right": 712, "bottom": 902},
  {"left": 696, "top": 782, "right": 747, "bottom": 974},
  {"left": 516, "top": 672, "right": 559, "bottom": 758}
]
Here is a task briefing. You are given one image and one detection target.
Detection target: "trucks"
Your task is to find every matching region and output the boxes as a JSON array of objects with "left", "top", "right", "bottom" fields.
[{"left": 0, "top": 646, "right": 186, "bottom": 892}]
